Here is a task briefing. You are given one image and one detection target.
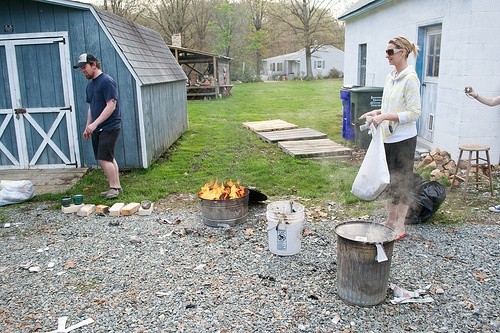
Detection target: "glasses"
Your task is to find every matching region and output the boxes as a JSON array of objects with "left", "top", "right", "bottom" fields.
[{"left": 386, "top": 49, "right": 402, "bottom": 55}]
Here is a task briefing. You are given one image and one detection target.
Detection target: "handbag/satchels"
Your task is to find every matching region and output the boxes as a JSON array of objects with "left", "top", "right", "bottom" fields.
[{"left": 350, "top": 124, "right": 391, "bottom": 201}]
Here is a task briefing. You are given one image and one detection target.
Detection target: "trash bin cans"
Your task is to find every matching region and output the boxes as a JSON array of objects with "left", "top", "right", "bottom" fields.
[
  {"left": 340, "top": 85, "right": 363, "bottom": 140},
  {"left": 348, "top": 86, "right": 384, "bottom": 149},
  {"left": 267, "top": 200, "right": 305, "bottom": 256},
  {"left": 335, "top": 221, "right": 397, "bottom": 304},
  {"left": 289, "top": 72, "right": 294, "bottom": 80}
]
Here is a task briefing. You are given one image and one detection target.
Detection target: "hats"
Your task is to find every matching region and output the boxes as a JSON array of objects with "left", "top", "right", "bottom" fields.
[{"left": 73, "top": 53, "right": 96, "bottom": 69}]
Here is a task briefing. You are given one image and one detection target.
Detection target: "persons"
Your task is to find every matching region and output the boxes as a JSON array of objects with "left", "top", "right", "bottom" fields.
[
  {"left": 73, "top": 53, "right": 123, "bottom": 199},
  {"left": 201, "top": 70, "right": 213, "bottom": 101},
  {"left": 464, "top": 85, "right": 500, "bottom": 213},
  {"left": 359, "top": 36, "right": 421, "bottom": 241}
]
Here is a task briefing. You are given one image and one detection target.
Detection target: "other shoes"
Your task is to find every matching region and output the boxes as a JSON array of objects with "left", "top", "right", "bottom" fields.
[
  {"left": 106, "top": 189, "right": 119, "bottom": 198},
  {"left": 393, "top": 227, "right": 406, "bottom": 240},
  {"left": 101, "top": 188, "right": 122, "bottom": 196}
]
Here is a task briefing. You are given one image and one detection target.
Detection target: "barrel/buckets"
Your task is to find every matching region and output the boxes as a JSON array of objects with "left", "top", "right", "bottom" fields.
[
  {"left": 333, "top": 221, "right": 396, "bottom": 306},
  {"left": 265, "top": 201, "right": 304, "bottom": 256},
  {"left": 198, "top": 188, "right": 250, "bottom": 227}
]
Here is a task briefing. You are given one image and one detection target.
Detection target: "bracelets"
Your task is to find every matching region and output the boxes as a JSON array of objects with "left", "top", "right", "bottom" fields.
[{"left": 474, "top": 93, "right": 478, "bottom": 98}]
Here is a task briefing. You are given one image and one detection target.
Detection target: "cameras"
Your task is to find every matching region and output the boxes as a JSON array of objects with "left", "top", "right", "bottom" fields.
[{"left": 465, "top": 87, "right": 472, "bottom": 93}]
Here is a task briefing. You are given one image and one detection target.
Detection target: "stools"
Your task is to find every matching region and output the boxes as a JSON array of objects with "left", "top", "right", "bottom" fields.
[{"left": 451, "top": 144, "right": 495, "bottom": 199}]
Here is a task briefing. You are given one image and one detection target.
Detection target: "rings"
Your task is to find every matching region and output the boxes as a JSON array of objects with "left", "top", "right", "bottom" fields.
[{"left": 375, "top": 125, "right": 377, "bottom": 126}]
